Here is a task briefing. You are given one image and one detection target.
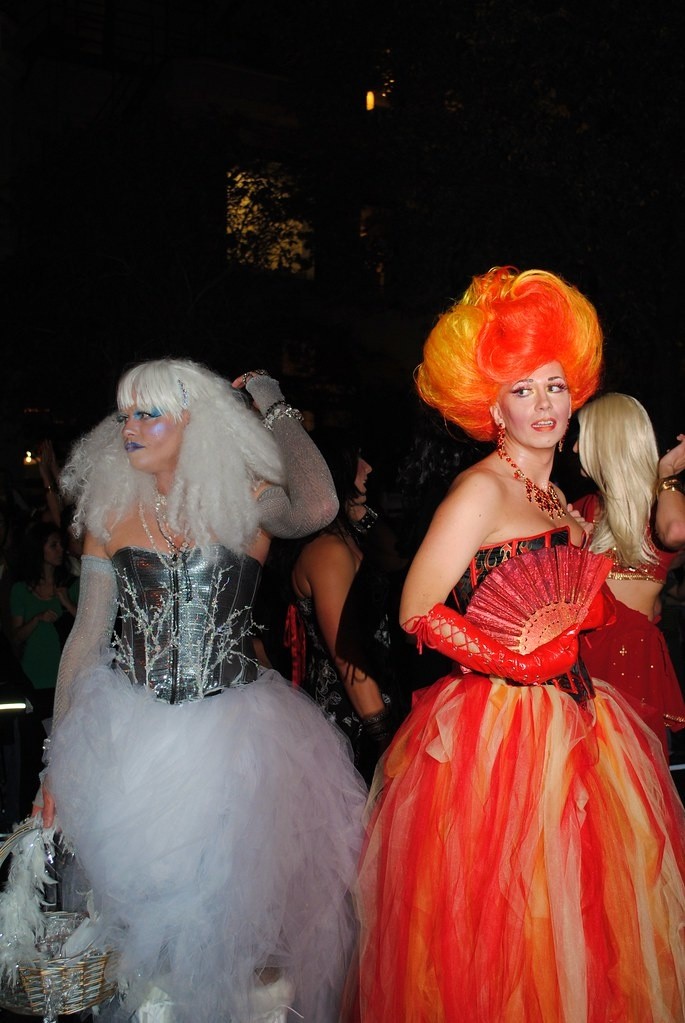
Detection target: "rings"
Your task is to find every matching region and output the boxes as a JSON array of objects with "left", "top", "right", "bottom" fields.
[
  {"left": 255, "top": 369, "right": 268, "bottom": 375},
  {"left": 243, "top": 372, "right": 253, "bottom": 384}
]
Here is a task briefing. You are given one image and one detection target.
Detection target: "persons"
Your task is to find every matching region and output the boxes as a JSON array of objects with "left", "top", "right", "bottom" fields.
[
  {"left": 342, "top": 265, "right": 685, "bottom": 1023},
  {"left": 0, "top": 438, "right": 86, "bottom": 890},
  {"left": 30, "top": 360, "right": 372, "bottom": 1023},
  {"left": 279, "top": 422, "right": 405, "bottom": 791},
  {"left": 567, "top": 394, "right": 685, "bottom": 736}
]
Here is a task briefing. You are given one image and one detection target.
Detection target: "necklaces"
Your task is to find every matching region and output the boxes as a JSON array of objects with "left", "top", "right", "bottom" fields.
[
  {"left": 495, "top": 441, "right": 566, "bottom": 520},
  {"left": 138, "top": 488, "right": 201, "bottom": 572}
]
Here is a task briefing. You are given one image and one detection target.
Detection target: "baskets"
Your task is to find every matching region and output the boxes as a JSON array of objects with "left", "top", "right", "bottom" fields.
[{"left": 0, "top": 818, "right": 120, "bottom": 1016}]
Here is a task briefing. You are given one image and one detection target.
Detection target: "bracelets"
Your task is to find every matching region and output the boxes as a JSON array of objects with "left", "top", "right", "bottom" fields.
[
  {"left": 656, "top": 475, "right": 683, "bottom": 498},
  {"left": 261, "top": 400, "right": 304, "bottom": 430}
]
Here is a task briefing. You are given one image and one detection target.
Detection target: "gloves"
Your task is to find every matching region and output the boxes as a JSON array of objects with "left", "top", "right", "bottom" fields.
[
  {"left": 578, "top": 586, "right": 617, "bottom": 632},
  {"left": 404, "top": 602, "right": 580, "bottom": 685}
]
